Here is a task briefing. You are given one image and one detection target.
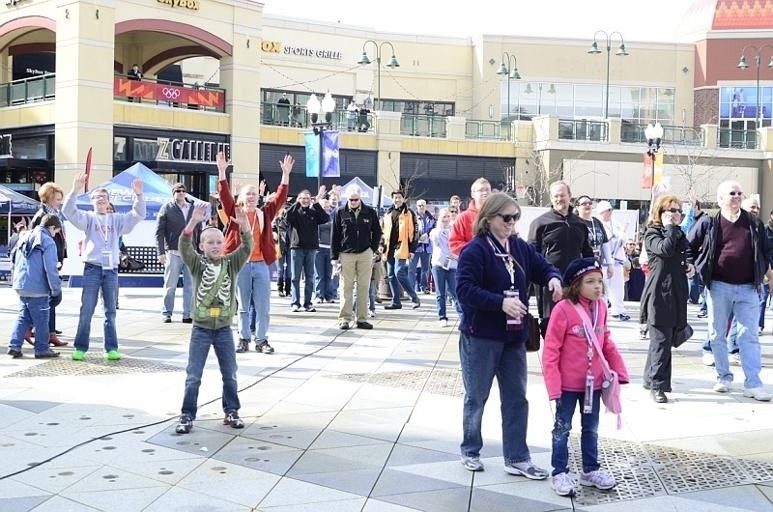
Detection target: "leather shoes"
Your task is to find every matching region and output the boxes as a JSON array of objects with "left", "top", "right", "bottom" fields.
[
  {"left": 385, "top": 304, "right": 401, "bottom": 309},
  {"left": 652, "top": 387, "right": 666, "bottom": 403},
  {"left": 412, "top": 298, "right": 420, "bottom": 308},
  {"left": 54, "top": 329, "right": 62, "bottom": 334},
  {"left": 643, "top": 381, "right": 649, "bottom": 387}
]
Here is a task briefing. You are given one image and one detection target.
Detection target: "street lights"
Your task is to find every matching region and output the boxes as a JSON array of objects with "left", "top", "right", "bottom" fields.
[
  {"left": 524, "top": 81, "right": 555, "bottom": 116},
  {"left": 588, "top": 30, "right": 629, "bottom": 119},
  {"left": 645, "top": 123, "right": 664, "bottom": 198},
  {"left": 496, "top": 52, "right": 522, "bottom": 121},
  {"left": 356, "top": 39, "right": 400, "bottom": 111},
  {"left": 736, "top": 44, "right": 773, "bottom": 130},
  {"left": 307, "top": 88, "right": 336, "bottom": 194}
]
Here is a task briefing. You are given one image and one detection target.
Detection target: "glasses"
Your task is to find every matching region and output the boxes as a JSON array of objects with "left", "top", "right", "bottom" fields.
[
  {"left": 449, "top": 211, "right": 456, "bottom": 213},
  {"left": 729, "top": 192, "right": 741, "bottom": 196},
  {"left": 663, "top": 208, "right": 682, "bottom": 213},
  {"left": 348, "top": 198, "right": 358, "bottom": 201},
  {"left": 578, "top": 203, "right": 591, "bottom": 206},
  {"left": 173, "top": 190, "right": 185, "bottom": 196},
  {"left": 629, "top": 243, "right": 634, "bottom": 245},
  {"left": 496, "top": 212, "right": 519, "bottom": 222}
]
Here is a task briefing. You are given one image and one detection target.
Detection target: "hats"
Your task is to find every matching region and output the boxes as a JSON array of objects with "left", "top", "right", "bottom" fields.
[
  {"left": 15, "top": 222, "right": 23, "bottom": 231},
  {"left": 596, "top": 200, "right": 612, "bottom": 214},
  {"left": 562, "top": 257, "right": 604, "bottom": 286}
]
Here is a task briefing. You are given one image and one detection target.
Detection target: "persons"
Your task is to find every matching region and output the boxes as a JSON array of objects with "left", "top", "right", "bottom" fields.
[
  {"left": 7, "top": 214, "right": 60, "bottom": 357},
  {"left": 687, "top": 199, "right": 710, "bottom": 317},
  {"left": 448, "top": 206, "right": 458, "bottom": 218},
  {"left": 448, "top": 178, "right": 492, "bottom": 256},
  {"left": 272, "top": 202, "right": 293, "bottom": 298},
  {"left": 625, "top": 239, "right": 640, "bottom": 261},
  {"left": 740, "top": 198, "right": 760, "bottom": 217},
  {"left": 575, "top": 195, "right": 614, "bottom": 280},
  {"left": 639, "top": 197, "right": 695, "bottom": 402},
  {"left": 757, "top": 210, "right": 773, "bottom": 336},
  {"left": 380, "top": 190, "right": 420, "bottom": 310},
  {"left": 258, "top": 180, "right": 266, "bottom": 206},
  {"left": 24, "top": 182, "right": 68, "bottom": 347},
  {"left": 450, "top": 195, "right": 461, "bottom": 209},
  {"left": 629, "top": 257, "right": 645, "bottom": 302},
  {"left": 318, "top": 185, "right": 342, "bottom": 219},
  {"left": 685, "top": 180, "right": 773, "bottom": 401},
  {"left": 127, "top": 64, "right": 144, "bottom": 102},
  {"left": 286, "top": 189, "right": 330, "bottom": 312},
  {"left": 105, "top": 202, "right": 127, "bottom": 309},
  {"left": 455, "top": 193, "right": 563, "bottom": 481},
  {"left": 541, "top": 257, "right": 629, "bottom": 497},
  {"left": 408, "top": 195, "right": 435, "bottom": 295},
  {"left": 313, "top": 198, "right": 339, "bottom": 304},
  {"left": 527, "top": 180, "right": 594, "bottom": 341},
  {"left": 595, "top": 200, "right": 630, "bottom": 321},
  {"left": 329, "top": 184, "right": 381, "bottom": 330},
  {"left": 175, "top": 203, "right": 253, "bottom": 433},
  {"left": 346, "top": 100, "right": 358, "bottom": 132},
  {"left": 276, "top": 93, "right": 290, "bottom": 127},
  {"left": 154, "top": 184, "right": 202, "bottom": 323},
  {"left": 61, "top": 172, "right": 147, "bottom": 361},
  {"left": 216, "top": 151, "right": 295, "bottom": 354},
  {"left": 430, "top": 208, "right": 462, "bottom": 327},
  {"left": 358, "top": 104, "right": 370, "bottom": 133}
]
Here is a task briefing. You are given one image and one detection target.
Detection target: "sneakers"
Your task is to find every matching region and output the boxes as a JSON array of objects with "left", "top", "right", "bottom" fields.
[
  {"left": 174, "top": 415, "right": 192, "bottom": 433},
  {"left": 182, "top": 318, "right": 191, "bottom": 323},
  {"left": 33, "top": 349, "right": 60, "bottom": 358},
  {"left": 221, "top": 411, "right": 244, "bottom": 428},
  {"left": 578, "top": 468, "right": 617, "bottom": 489},
  {"left": 107, "top": 350, "right": 119, "bottom": 360},
  {"left": 71, "top": 350, "right": 84, "bottom": 360},
  {"left": 351, "top": 311, "right": 354, "bottom": 319},
  {"left": 459, "top": 452, "right": 483, "bottom": 470},
  {"left": 620, "top": 314, "right": 630, "bottom": 321},
  {"left": 285, "top": 293, "right": 291, "bottom": 297},
  {"left": 440, "top": 319, "right": 446, "bottom": 326},
  {"left": 371, "top": 309, "right": 375, "bottom": 317},
  {"left": 162, "top": 315, "right": 171, "bottom": 323},
  {"left": 743, "top": 386, "right": 773, "bottom": 401},
  {"left": 550, "top": 472, "right": 576, "bottom": 495},
  {"left": 357, "top": 323, "right": 372, "bottom": 329},
  {"left": 338, "top": 321, "right": 348, "bottom": 329},
  {"left": 256, "top": 339, "right": 273, "bottom": 353},
  {"left": 316, "top": 299, "right": 320, "bottom": 303},
  {"left": 327, "top": 299, "right": 334, "bottom": 303},
  {"left": 7, "top": 349, "right": 22, "bottom": 357},
  {"left": 713, "top": 379, "right": 729, "bottom": 392},
  {"left": 305, "top": 308, "right": 315, "bottom": 312},
  {"left": 503, "top": 460, "right": 548, "bottom": 480},
  {"left": 291, "top": 305, "right": 298, "bottom": 311},
  {"left": 236, "top": 339, "right": 248, "bottom": 352},
  {"left": 279, "top": 291, "right": 284, "bottom": 297}
]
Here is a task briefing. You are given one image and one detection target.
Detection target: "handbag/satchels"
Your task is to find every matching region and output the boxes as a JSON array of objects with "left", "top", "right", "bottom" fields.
[
  {"left": 567, "top": 296, "right": 621, "bottom": 429},
  {"left": 487, "top": 234, "right": 540, "bottom": 352}
]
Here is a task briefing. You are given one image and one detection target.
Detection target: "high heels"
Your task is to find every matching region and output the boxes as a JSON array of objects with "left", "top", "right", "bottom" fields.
[
  {"left": 47, "top": 332, "right": 68, "bottom": 346},
  {"left": 23, "top": 326, "right": 34, "bottom": 345}
]
